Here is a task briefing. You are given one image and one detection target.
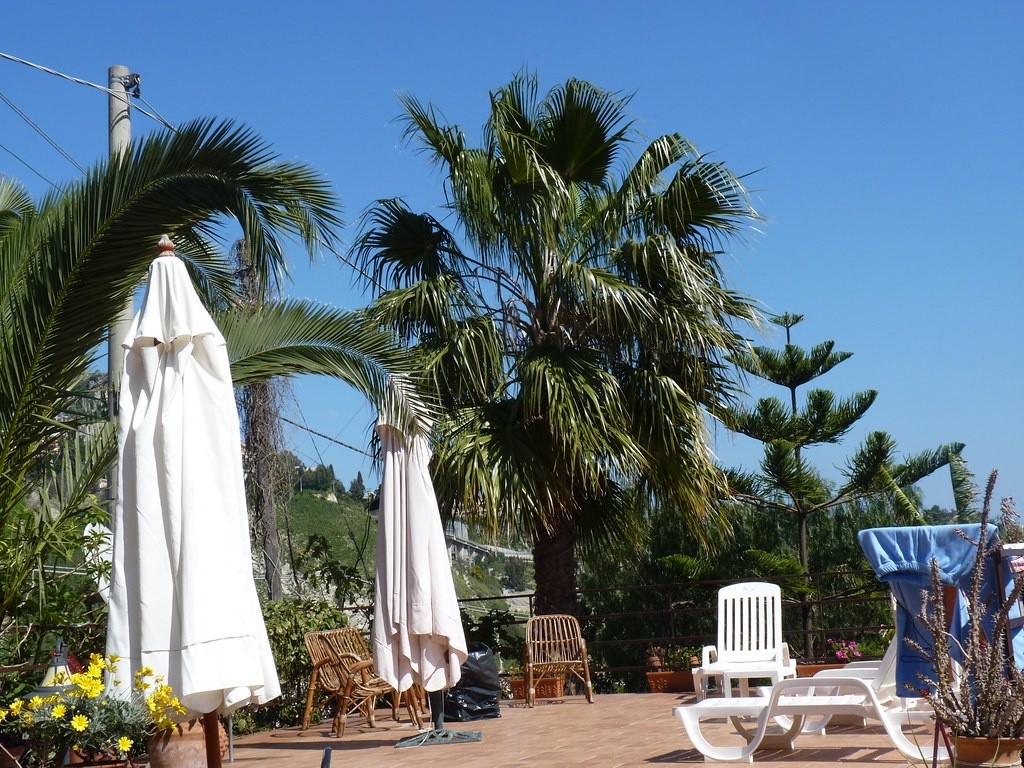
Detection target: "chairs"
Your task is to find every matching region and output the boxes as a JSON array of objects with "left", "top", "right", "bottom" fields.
[
  {"left": 320, "top": 636, "right": 425, "bottom": 738},
  {"left": 524, "top": 613, "right": 595, "bottom": 708},
  {"left": 304, "top": 628, "right": 425, "bottom": 734},
  {"left": 677, "top": 522, "right": 1024, "bottom": 760}
]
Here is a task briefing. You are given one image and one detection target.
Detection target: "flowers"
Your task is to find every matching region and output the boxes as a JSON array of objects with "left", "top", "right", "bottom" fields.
[
  {"left": 0, "top": 652, "right": 187, "bottom": 752},
  {"left": 827, "top": 638, "right": 862, "bottom": 662}
]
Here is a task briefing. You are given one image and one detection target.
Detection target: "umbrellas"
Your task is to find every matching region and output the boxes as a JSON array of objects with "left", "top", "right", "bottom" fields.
[
  {"left": 102, "top": 235, "right": 285, "bottom": 768},
  {"left": 369, "top": 372, "right": 468, "bottom": 733}
]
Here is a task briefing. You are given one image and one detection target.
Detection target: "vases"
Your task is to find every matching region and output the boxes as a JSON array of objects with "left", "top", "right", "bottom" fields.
[
  {"left": 69, "top": 735, "right": 140, "bottom": 768},
  {"left": 797, "top": 657, "right": 846, "bottom": 677},
  {"left": 0, "top": 745, "right": 25, "bottom": 768}
]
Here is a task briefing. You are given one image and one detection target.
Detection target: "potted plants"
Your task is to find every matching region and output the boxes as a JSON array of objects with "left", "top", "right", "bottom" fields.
[
  {"left": 904, "top": 468, "right": 1024, "bottom": 768},
  {"left": 645, "top": 646, "right": 695, "bottom": 693},
  {"left": 645, "top": 644, "right": 664, "bottom": 670},
  {"left": 688, "top": 646, "right": 703, "bottom": 667}
]
